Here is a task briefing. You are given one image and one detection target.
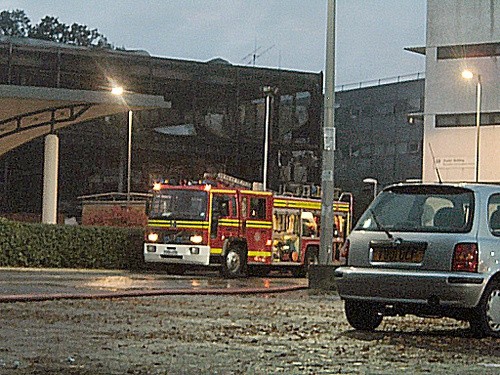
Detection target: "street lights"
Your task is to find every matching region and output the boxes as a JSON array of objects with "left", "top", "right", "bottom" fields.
[
  {"left": 463, "top": 70, "right": 481, "bottom": 182},
  {"left": 363, "top": 177, "right": 378, "bottom": 200},
  {"left": 110, "top": 84, "right": 133, "bottom": 201}
]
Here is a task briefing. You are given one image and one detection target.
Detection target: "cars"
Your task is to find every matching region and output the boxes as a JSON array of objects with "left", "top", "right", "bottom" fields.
[{"left": 334, "top": 178, "right": 499, "bottom": 338}]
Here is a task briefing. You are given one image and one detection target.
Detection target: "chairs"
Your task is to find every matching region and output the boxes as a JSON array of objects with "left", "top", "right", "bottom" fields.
[{"left": 433, "top": 207, "right": 464, "bottom": 229}]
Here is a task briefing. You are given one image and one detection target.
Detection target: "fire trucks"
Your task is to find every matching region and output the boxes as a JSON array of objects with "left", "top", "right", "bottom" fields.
[{"left": 144, "top": 183, "right": 353, "bottom": 279}]
